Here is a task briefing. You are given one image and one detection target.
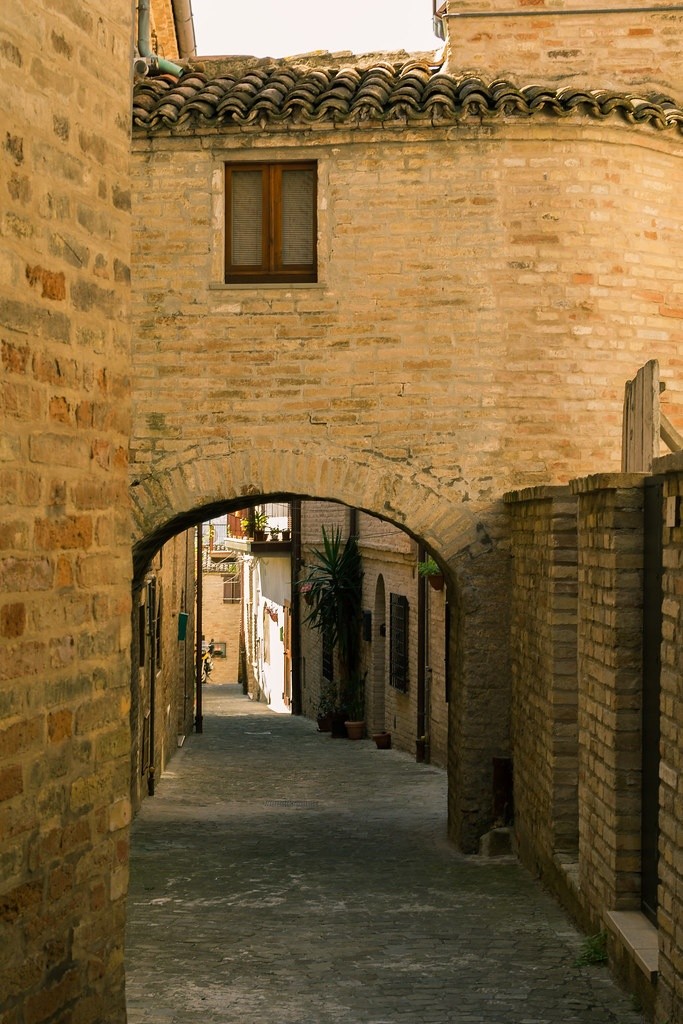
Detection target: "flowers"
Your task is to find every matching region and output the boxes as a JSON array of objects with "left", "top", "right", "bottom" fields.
[{"left": 299, "top": 583, "right": 313, "bottom": 593}]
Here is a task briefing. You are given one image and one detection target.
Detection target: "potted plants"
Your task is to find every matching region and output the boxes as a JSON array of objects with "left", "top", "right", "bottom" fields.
[
  {"left": 241, "top": 512, "right": 268, "bottom": 541},
  {"left": 270, "top": 528, "right": 282, "bottom": 540},
  {"left": 315, "top": 683, "right": 337, "bottom": 732},
  {"left": 330, "top": 689, "right": 349, "bottom": 738},
  {"left": 417, "top": 557, "right": 444, "bottom": 591},
  {"left": 282, "top": 528, "right": 291, "bottom": 540},
  {"left": 343, "top": 669, "right": 368, "bottom": 740}
]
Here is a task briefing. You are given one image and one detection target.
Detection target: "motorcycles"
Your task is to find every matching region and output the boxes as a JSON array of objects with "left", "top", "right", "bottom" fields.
[{"left": 193, "top": 639, "right": 215, "bottom": 684}]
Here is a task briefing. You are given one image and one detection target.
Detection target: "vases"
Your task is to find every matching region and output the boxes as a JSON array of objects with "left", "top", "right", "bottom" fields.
[{"left": 303, "top": 590, "right": 314, "bottom": 605}]
